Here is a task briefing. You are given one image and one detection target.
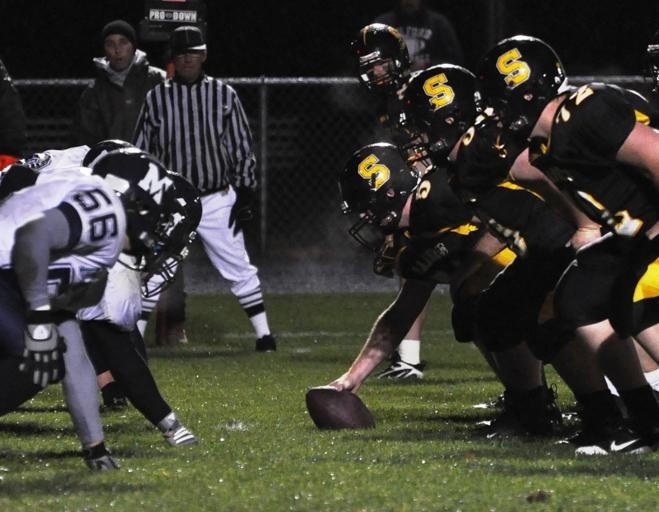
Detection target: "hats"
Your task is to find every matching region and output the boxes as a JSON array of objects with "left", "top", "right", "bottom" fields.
[
  {"left": 101, "top": 20, "right": 136, "bottom": 56},
  {"left": 172, "top": 25, "right": 207, "bottom": 54}
]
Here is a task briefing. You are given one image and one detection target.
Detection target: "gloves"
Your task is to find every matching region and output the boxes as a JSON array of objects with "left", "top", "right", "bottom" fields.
[
  {"left": 228, "top": 187, "right": 257, "bottom": 238},
  {"left": 17, "top": 311, "right": 67, "bottom": 388},
  {"left": 83, "top": 442, "right": 117, "bottom": 471}
]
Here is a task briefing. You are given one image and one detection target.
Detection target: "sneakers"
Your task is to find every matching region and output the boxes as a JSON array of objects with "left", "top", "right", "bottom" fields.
[
  {"left": 159, "top": 413, "right": 197, "bottom": 447},
  {"left": 490, "top": 385, "right": 562, "bottom": 435},
  {"left": 374, "top": 351, "right": 427, "bottom": 379},
  {"left": 575, "top": 423, "right": 659, "bottom": 455},
  {"left": 553, "top": 411, "right": 628, "bottom": 445},
  {"left": 255, "top": 334, "right": 276, "bottom": 352}
]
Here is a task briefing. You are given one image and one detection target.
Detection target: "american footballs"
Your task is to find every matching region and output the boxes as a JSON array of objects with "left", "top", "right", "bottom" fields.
[{"left": 305, "top": 386, "right": 374, "bottom": 430}]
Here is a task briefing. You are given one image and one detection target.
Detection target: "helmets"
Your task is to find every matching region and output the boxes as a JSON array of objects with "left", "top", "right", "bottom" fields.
[
  {"left": 472, "top": 34, "right": 567, "bottom": 139},
  {"left": 351, "top": 23, "right": 409, "bottom": 97},
  {"left": 396, "top": 64, "right": 487, "bottom": 179},
  {"left": 338, "top": 141, "right": 419, "bottom": 253},
  {"left": 82, "top": 139, "right": 134, "bottom": 167},
  {"left": 641, "top": 28, "right": 659, "bottom": 97},
  {"left": 92, "top": 147, "right": 177, "bottom": 263},
  {"left": 140, "top": 170, "right": 201, "bottom": 297}
]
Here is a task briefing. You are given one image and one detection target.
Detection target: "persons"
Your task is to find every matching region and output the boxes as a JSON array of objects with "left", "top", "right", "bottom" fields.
[
  {"left": 308, "top": 18, "right": 659, "bottom": 455},
  {"left": 69, "top": 21, "right": 277, "bottom": 353},
  {"left": 1, "top": 139, "right": 202, "bottom": 470},
  {"left": 1, "top": 58, "right": 34, "bottom": 161}
]
